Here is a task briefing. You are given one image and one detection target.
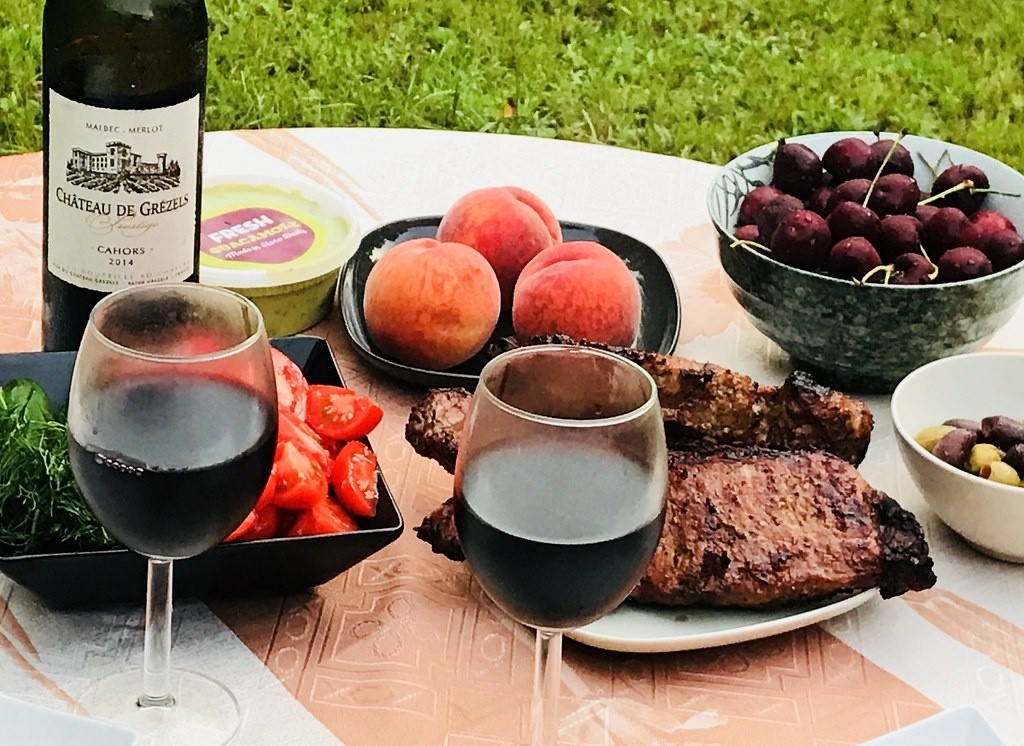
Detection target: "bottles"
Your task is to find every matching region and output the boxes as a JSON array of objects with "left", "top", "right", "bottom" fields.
[{"left": 42, "top": 0, "right": 210, "bottom": 350}]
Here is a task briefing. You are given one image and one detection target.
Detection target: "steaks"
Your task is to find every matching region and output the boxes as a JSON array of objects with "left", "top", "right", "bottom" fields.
[{"left": 404, "top": 332, "right": 938, "bottom": 608}]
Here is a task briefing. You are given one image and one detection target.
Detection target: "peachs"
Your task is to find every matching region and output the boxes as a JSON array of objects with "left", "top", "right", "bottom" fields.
[{"left": 362, "top": 182, "right": 643, "bottom": 370}]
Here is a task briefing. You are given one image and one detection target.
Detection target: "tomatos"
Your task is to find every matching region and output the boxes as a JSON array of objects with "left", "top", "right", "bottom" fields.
[{"left": 136, "top": 335, "right": 386, "bottom": 545}]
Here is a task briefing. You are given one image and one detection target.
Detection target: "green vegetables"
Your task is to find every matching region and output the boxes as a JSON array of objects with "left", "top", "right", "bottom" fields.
[{"left": 0, "top": 376, "right": 124, "bottom": 557}]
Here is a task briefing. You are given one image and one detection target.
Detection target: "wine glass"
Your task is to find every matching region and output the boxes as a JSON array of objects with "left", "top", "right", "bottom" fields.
[
  {"left": 452, "top": 344, "right": 669, "bottom": 746},
  {"left": 66, "top": 280, "right": 279, "bottom": 746}
]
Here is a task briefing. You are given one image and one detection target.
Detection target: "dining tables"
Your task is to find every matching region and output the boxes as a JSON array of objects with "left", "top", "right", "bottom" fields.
[{"left": 0, "top": 125, "right": 1024, "bottom": 746}]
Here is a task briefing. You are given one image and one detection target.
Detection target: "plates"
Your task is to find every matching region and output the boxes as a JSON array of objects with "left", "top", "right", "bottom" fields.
[
  {"left": 337, "top": 213, "right": 683, "bottom": 388},
  {"left": 0, "top": 332, "right": 403, "bottom": 611},
  {"left": 562, "top": 586, "right": 877, "bottom": 653}
]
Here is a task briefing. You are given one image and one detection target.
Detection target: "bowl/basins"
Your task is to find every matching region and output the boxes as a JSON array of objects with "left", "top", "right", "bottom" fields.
[
  {"left": 892, "top": 352, "right": 1024, "bottom": 567},
  {"left": 707, "top": 129, "right": 1024, "bottom": 377}
]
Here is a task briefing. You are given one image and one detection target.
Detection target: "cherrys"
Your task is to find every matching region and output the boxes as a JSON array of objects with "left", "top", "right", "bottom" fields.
[{"left": 729, "top": 130, "right": 1024, "bottom": 285}]
